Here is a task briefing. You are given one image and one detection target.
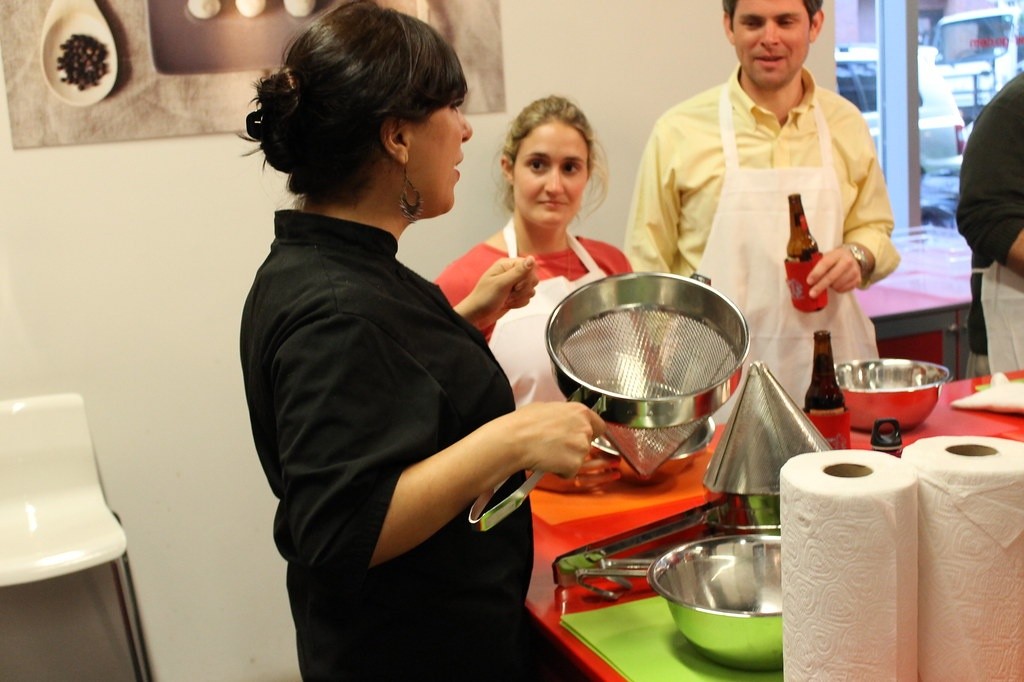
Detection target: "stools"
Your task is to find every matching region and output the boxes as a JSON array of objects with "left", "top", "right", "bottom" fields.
[{"left": 0, "top": 396, "right": 154, "bottom": 682}]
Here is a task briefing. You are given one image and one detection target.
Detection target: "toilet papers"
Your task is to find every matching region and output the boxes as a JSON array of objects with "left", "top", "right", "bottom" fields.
[
  {"left": 900, "top": 435, "right": 1024, "bottom": 682},
  {"left": 778, "top": 449, "right": 918, "bottom": 682}
]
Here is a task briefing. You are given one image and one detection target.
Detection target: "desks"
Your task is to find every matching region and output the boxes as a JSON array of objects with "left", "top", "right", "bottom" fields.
[
  {"left": 519, "top": 368, "right": 1023, "bottom": 682},
  {"left": 850, "top": 224, "right": 974, "bottom": 383}
]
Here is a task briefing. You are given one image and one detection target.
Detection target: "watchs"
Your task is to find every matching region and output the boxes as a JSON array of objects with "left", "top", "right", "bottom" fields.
[{"left": 847, "top": 243, "right": 867, "bottom": 272}]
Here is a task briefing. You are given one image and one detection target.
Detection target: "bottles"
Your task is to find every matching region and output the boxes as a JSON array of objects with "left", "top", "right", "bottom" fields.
[
  {"left": 786, "top": 193, "right": 828, "bottom": 312},
  {"left": 803, "top": 330, "right": 852, "bottom": 453}
]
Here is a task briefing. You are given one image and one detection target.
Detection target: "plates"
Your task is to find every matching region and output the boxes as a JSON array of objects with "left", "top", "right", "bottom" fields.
[{"left": 40, "top": 0, "right": 118, "bottom": 108}]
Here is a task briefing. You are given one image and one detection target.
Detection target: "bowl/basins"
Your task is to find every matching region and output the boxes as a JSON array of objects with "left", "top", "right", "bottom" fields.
[
  {"left": 646, "top": 534, "right": 784, "bottom": 671},
  {"left": 832, "top": 359, "right": 949, "bottom": 434}
]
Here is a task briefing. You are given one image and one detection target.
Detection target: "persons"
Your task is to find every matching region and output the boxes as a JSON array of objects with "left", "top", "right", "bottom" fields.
[
  {"left": 238, "top": 1, "right": 605, "bottom": 682},
  {"left": 956, "top": 71, "right": 1024, "bottom": 377},
  {"left": 436, "top": 94, "right": 635, "bottom": 493},
  {"left": 626, "top": 1, "right": 900, "bottom": 424}
]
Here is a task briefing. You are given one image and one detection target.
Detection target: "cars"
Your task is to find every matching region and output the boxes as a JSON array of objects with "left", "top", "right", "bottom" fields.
[
  {"left": 930, "top": 10, "right": 1024, "bottom": 110},
  {"left": 834, "top": 43, "right": 968, "bottom": 178}
]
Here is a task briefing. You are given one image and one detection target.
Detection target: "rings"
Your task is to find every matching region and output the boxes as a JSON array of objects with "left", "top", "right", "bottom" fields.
[{"left": 573, "top": 476, "right": 581, "bottom": 488}]
